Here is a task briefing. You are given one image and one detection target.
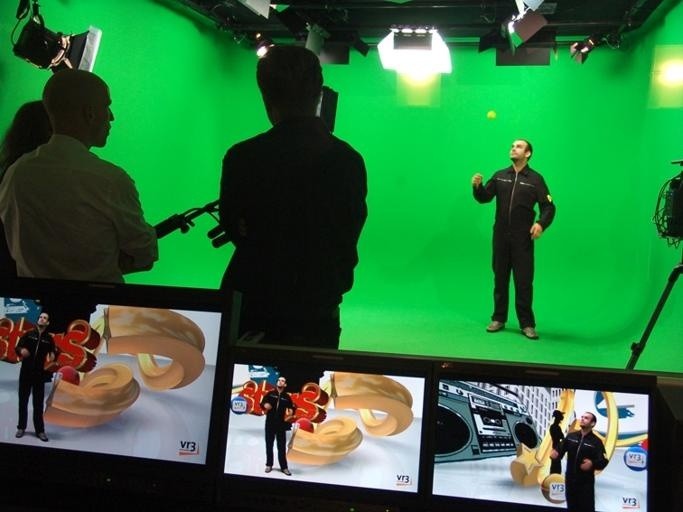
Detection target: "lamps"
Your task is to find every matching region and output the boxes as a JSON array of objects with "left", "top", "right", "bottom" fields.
[
  {"left": 296, "top": 7, "right": 349, "bottom": 64},
  {"left": 11, "top": 0, "right": 101, "bottom": 73},
  {"left": 499, "top": 0, "right": 548, "bottom": 48},
  {"left": 375, "top": 10, "right": 437, "bottom": 49},
  {"left": 569, "top": 28, "right": 611, "bottom": 64},
  {"left": 246, "top": 30, "right": 277, "bottom": 58}
]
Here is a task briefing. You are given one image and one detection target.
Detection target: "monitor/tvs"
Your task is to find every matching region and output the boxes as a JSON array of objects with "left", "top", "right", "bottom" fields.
[
  {"left": 427, "top": 348, "right": 657, "bottom": 512},
  {"left": 0, "top": 277, "right": 243, "bottom": 506},
  {"left": 216, "top": 333, "right": 432, "bottom": 512}
]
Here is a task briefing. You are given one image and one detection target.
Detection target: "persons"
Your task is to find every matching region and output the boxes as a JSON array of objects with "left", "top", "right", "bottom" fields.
[
  {"left": 0, "top": 100, "right": 50, "bottom": 280},
  {"left": 0, "top": 68, "right": 159, "bottom": 285},
  {"left": 548, "top": 411, "right": 610, "bottom": 512},
  {"left": 471, "top": 137, "right": 556, "bottom": 340},
  {"left": 547, "top": 409, "right": 568, "bottom": 474},
  {"left": 218, "top": 45, "right": 369, "bottom": 351},
  {"left": 13, "top": 308, "right": 55, "bottom": 443},
  {"left": 258, "top": 373, "right": 294, "bottom": 476}
]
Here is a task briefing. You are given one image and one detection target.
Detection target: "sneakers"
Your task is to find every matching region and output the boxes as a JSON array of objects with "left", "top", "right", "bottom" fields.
[
  {"left": 265, "top": 465, "right": 272, "bottom": 473},
  {"left": 522, "top": 326, "right": 538, "bottom": 339},
  {"left": 487, "top": 321, "right": 505, "bottom": 332},
  {"left": 35, "top": 432, "right": 49, "bottom": 441},
  {"left": 282, "top": 468, "right": 291, "bottom": 476},
  {"left": 16, "top": 429, "right": 24, "bottom": 438}
]
